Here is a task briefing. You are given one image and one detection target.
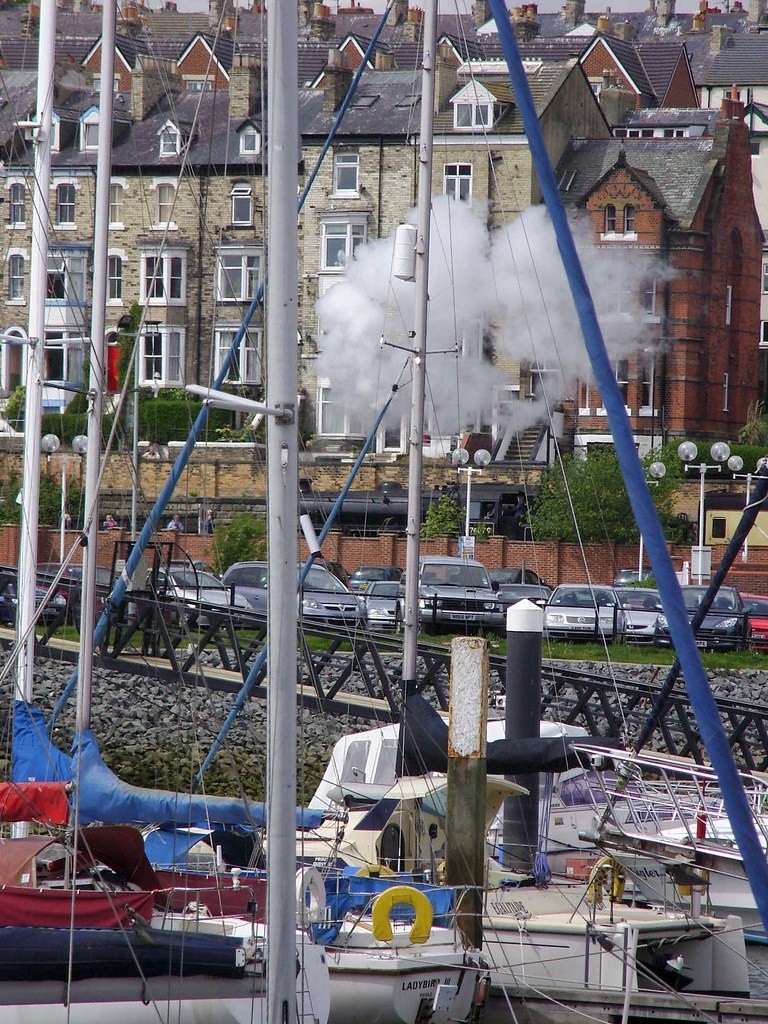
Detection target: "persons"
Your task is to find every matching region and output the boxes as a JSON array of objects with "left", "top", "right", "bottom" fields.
[
  {"left": 204, "top": 509, "right": 215, "bottom": 534},
  {"left": 166, "top": 515, "right": 184, "bottom": 533},
  {"left": 103, "top": 515, "right": 117, "bottom": 531},
  {"left": 64, "top": 514, "right": 74, "bottom": 530}
]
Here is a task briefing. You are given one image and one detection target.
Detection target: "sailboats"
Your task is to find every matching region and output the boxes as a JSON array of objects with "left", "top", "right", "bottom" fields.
[{"left": 0, "top": 0, "right": 767, "bottom": 1024}]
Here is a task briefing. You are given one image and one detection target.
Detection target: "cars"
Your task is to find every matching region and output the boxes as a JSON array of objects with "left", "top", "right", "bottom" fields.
[
  {"left": 536, "top": 583, "right": 632, "bottom": 644},
  {"left": 312, "top": 559, "right": 351, "bottom": 589},
  {"left": 0, "top": 563, "right": 66, "bottom": 628},
  {"left": 483, "top": 568, "right": 553, "bottom": 594},
  {"left": 612, "top": 568, "right": 659, "bottom": 589},
  {"left": 161, "top": 560, "right": 223, "bottom": 582},
  {"left": 344, "top": 564, "right": 401, "bottom": 594},
  {"left": 610, "top": 586, "right": 673, "bottom": 648},
  {"left": 738, "top": 592, "right": 767, "bottom": 654},
  {"left": 354, "top": 579, "right": 401, "bottom": 635},
  {"left": 125, "top": 567, "right": 256, "bottom": 633},
  {"left": 678, "top": 583, "right": 751, "bottom": 652},
  {"left": 36, "top": 563, "right": 112, "bottom": 604},
  {"left": 499, "top": 584, "right": 553, "bottom": 638}
]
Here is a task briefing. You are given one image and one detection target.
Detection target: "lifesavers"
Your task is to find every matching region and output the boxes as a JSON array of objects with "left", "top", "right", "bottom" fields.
[
  {"left": 355, "top": 865, "right": 395, "bottom": 878},
  {"left": 677, "top": 867, "right": 709, "bottom": 898},
  {"left": 371, "top": 885, "right": 435, "bottom": 943},
  {"left": 587, "top": 855, "right": 626, "bottom": 906},
  {"left": 295, "top": 866, "right": 327, "bottom": 925}
]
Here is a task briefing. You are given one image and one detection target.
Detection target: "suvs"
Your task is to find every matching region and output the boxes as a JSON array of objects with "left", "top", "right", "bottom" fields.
[
  {"left": 218, "top": 561, "right": 368, "bottom": 631},
  {"left": 398, "top": 555, "right": 504, "bottom": 638}
]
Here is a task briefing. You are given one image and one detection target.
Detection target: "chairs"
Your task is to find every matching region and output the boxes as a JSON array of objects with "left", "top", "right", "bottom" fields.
[
  {"left": 643, "top": 598, "right": 660, "bottom": 608},
  {"left": 596, "top": 594, "right": 610, "bottom": 606},
  {"left": 424, "top": 572, "right": 436, "bottom": 583},
  {"left": 716, "top": 597, "right": 733, "bottom": 610}
]
